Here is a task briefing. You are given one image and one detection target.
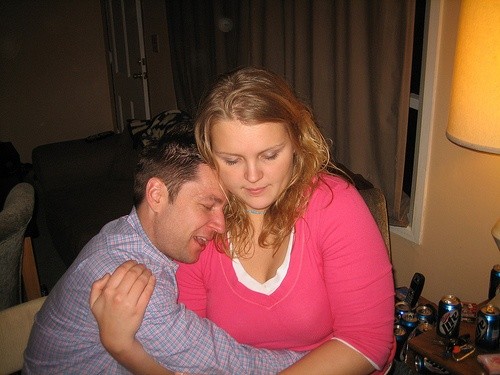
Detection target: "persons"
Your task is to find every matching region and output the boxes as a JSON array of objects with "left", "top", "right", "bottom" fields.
[
  {"left": 24, "top": 140, "right": 314, "bottom": 374},
  {"left": 89, "top": 66, "right": 399, "bottom": 375}
]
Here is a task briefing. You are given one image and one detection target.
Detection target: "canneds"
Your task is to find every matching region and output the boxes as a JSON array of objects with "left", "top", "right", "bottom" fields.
[
  {"left": 394, "top": 303, "right": 454, "bottom": 375},
  {"left": 489, "top": 264, "right": 500, "bottom": 301},
  {"left": 474, "top": 304, "right": 500, "bottom": 353},
  {"left": 436, "top": 295, "right": 462, "bottom": 338}
]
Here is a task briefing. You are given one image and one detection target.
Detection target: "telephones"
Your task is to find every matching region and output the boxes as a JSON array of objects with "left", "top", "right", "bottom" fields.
[{"left": 405, "top": 272, "right": 425, "bottom": 305}]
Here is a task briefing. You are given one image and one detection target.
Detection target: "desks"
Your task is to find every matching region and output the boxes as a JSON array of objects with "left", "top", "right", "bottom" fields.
[{"left": 408, "top": 296, "right": 500, "bottom": 375}]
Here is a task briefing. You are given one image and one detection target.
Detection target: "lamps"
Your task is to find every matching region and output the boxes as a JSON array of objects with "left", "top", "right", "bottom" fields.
[{"left": 445, "top": 0, "right": 500, "bottom": 258}]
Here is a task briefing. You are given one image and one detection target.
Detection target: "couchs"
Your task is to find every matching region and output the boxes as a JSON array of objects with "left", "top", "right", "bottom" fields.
[{"left": 0, "top": 130, "right": 131, "bottom": 310}]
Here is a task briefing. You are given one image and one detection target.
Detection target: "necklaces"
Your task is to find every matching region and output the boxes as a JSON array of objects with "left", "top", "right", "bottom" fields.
[{"left": 241, "top": 209, "right": 268, "bottom": 214}]
